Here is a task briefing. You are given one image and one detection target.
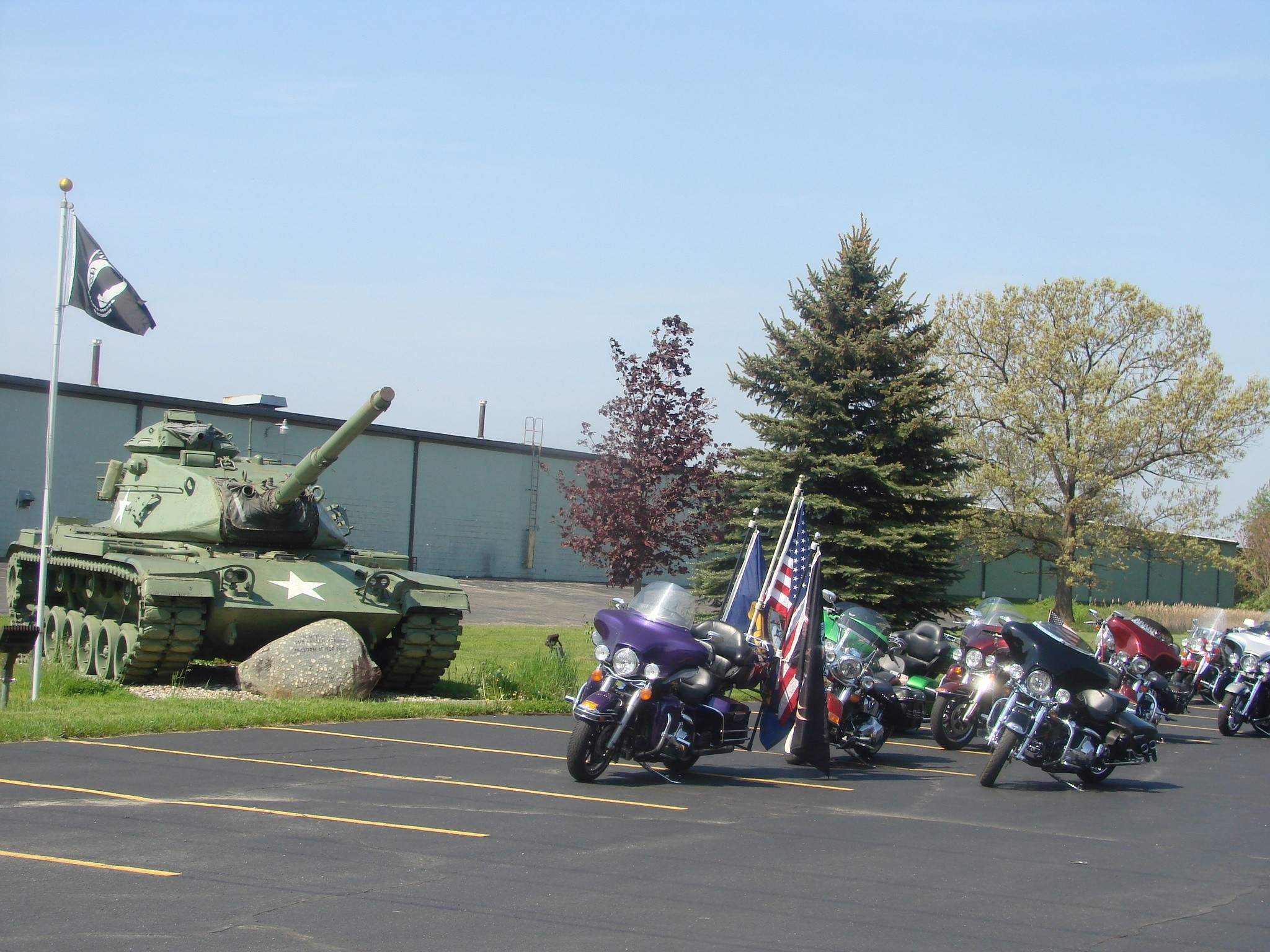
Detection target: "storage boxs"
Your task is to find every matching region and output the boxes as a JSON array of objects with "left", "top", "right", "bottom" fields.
[
  {"left": 732, "top": 651, "right": 767, "bottom": 690},
  {"left": 939, "top": 645, "right": 960, "bottom": 674}
]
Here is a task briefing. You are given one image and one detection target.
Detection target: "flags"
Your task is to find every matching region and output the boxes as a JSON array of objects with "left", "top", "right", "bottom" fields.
[
  {"left": 760, "top": 498, "right": 830, "bottom": 778},
  {"left": 59, "top": 211, "right": 156, "bottom": 336},
  {"left": 720, "top": 507, "right": 783, "bottom": 663}
]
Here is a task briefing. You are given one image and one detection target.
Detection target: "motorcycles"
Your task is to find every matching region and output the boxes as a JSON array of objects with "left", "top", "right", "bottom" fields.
[
  {"left": 980, "top": 607, "right": 1164, "bottom": 789},
  {"left": 563, "top": 582, "right": 760, "bottom": 783},
  {"left": 779, "top": 585, "right": 1270, "bottom": 765}
]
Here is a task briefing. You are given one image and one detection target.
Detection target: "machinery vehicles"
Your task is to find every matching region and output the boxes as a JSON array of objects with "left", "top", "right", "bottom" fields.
[{"left": 0, "top": 384, "right": 472, "bottom": 696}]
